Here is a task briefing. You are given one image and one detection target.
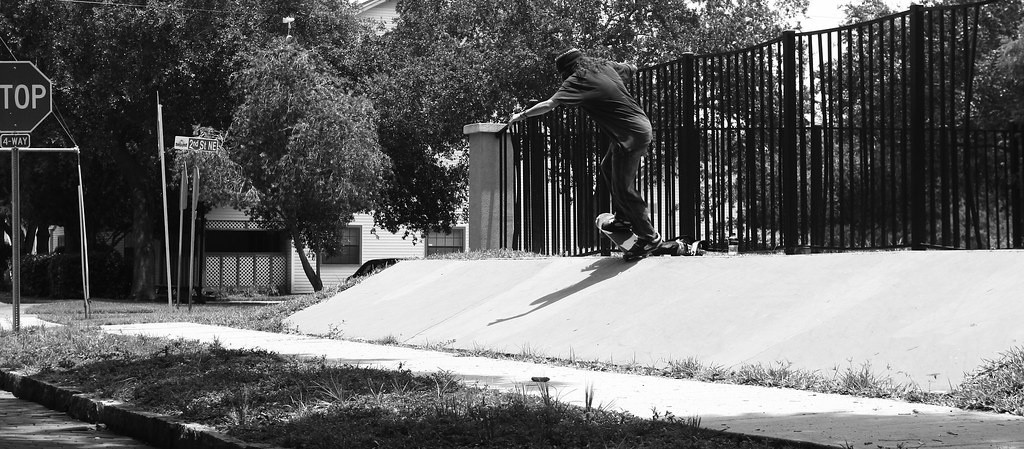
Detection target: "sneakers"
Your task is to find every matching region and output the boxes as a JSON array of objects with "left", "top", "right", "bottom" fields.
[
  {"left": 601, "top": 218, "right": 635, "bottom": 232},
  {"left": 623, "top": 232, "right": 663, "bottom": 261}
]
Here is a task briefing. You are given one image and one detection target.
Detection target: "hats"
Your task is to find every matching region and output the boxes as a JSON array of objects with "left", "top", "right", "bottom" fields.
[{"left": 555, "top": 46, "right": 585, "bottom": 75}]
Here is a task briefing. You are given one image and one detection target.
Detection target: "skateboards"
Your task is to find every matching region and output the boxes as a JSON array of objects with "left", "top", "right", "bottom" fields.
[{"left": 594, "top": 212, "right": 649, "bottom": 261}]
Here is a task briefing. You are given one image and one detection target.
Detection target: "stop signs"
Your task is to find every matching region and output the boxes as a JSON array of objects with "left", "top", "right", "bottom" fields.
[{"left": 0, "top": 62, "right": 52, "bottom": 133}]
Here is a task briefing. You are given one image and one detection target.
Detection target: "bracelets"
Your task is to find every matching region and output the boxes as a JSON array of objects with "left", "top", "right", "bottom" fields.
[{"left": 520, "top": 110, "right": 528, "bottom": 119}]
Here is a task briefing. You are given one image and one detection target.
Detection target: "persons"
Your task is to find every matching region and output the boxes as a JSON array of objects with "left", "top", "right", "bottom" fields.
[{"left": 508, "top": 47, "right": 664, "bottom": 259}]
